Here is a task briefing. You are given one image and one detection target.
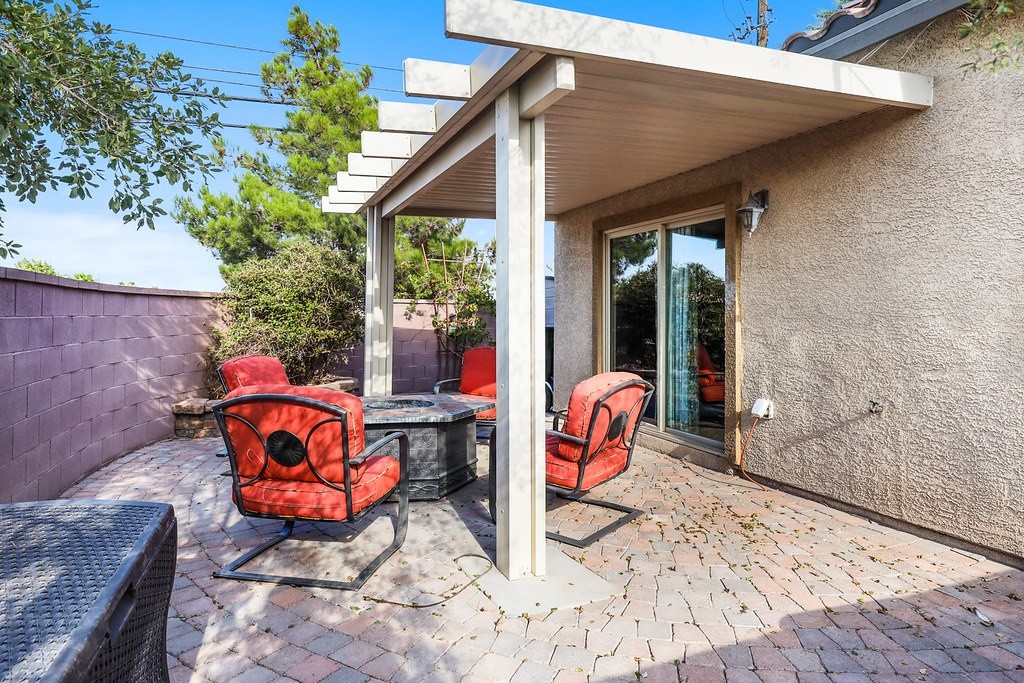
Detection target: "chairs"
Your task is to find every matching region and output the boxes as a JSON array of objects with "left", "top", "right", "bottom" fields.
[
  {"left": 212, "top": 384, "right": 410, "bottom": 591},
  {"left": 697, "top": 346, "right": 725, "bottom": 422},
  {"left": 488, "top": 373, "right": 656, "bottom": 548},
  {"left": 433, "top": 347, "right": 497, "bottom": 438},
  {"left": 215, "top": 355, "right": 290, "bottom": 395}
]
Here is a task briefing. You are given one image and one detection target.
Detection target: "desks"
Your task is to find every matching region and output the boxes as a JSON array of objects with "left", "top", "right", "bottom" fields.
[{"left": 0, "top": 500, "right": 177, "bottom": 683}]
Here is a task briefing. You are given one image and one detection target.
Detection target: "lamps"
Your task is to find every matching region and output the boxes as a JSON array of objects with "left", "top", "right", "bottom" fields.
[{"left": 736, "top": 189, "right": 769, "bottom": 239}]
